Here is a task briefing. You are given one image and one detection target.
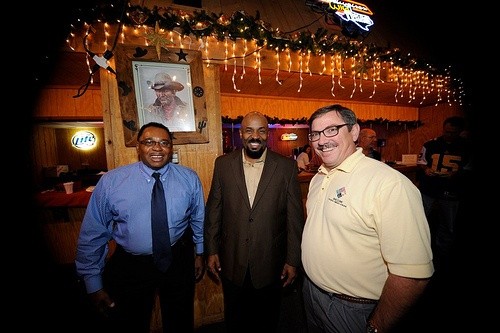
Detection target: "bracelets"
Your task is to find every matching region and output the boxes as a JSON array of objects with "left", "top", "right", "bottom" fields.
[{"left": 365, "top": 320, "right": 382, "bottom": 333}]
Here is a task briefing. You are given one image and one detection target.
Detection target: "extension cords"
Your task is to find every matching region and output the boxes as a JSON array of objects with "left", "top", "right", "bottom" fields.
[{"left": 93, "top": 55, "right": 109, "bottom": 69}]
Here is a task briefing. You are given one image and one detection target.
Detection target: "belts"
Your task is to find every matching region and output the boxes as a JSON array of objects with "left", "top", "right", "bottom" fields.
[{"left": 333, "top": 294, "right": 378, "bottom": 303}]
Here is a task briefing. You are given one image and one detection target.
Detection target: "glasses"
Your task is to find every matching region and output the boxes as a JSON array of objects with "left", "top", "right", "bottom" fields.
[
  {"left": 139, "top": 139, "right": 171, "bottom": 147},
  {"left": 307, "top": 124, "right": 349, "bottom": 142}
]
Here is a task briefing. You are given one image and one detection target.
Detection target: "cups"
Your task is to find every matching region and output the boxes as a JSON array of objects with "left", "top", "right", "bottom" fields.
[{"left": 63, "top": 182, "right": 74, "bottom": 194}]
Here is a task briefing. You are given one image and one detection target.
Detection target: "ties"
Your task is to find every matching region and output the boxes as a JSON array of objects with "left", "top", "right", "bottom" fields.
[{"left": 151, "top": 173, "right": 171, "bottom": 271}]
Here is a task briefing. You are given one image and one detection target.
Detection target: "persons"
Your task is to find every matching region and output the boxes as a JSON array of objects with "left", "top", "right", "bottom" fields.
[
  {"left": 203, "top": 111, "right": 304, "bottom": 333},
  {"left": 75, "top": 123, "right": 205, "bottom": 333},
  {"left": 355, "top": 117, "right": 477, "bottom": 239},
  {"left": 301, "top": 105, "right": 435, "bottom": 333},
  {"left": 297, "top": 144, "right": 311, "bottom": 171}
]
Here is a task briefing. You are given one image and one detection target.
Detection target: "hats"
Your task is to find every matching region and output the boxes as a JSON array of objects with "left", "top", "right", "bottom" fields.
[{"left": 147, "top": 73, "right": 184, "bottom": 91}]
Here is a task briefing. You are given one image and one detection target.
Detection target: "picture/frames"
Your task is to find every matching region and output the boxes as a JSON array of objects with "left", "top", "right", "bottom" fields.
[{"left": 113, "top": 41, "right": 210, "bottom": 147}]
